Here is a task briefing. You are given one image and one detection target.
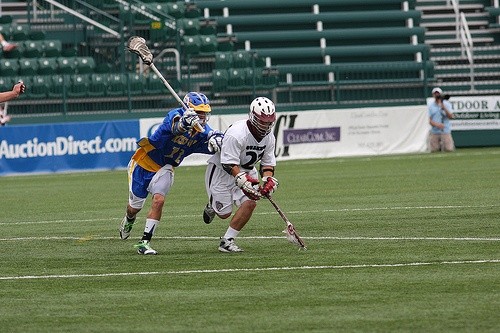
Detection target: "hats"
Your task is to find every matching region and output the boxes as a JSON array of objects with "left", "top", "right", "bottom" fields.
[{"left": 432, "top": 87, "right": 443, "bottom": 94}]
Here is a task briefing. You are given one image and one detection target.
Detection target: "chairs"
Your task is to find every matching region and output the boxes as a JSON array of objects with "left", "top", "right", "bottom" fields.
[{"left": 0, "top": 0, "right": 281, "bottom": 101}]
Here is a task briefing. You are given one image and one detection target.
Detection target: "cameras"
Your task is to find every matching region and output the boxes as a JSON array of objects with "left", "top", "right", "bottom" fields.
[{"left": 433, "top": 95, "right": 450, "bottom": 103}]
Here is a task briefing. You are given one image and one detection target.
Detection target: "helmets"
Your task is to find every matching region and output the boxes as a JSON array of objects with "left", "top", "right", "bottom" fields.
[
  {"left": 183, "top": 92, "right": 211, "bottom": 126},
  {"left": 250, "top": 97, "right": 275, "bottom": 136}
]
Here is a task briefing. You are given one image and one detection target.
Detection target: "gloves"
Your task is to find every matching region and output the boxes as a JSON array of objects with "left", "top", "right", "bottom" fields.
[
  {"left": 208, "top": 130, "right": 224, "bottom": 152},
  {"left": 178, "top": 108, "right": 200, "bottom": 131},
  {"left": 262, "top": 176, "right": 279, "bottom": 197},
  {"left": 235, "top": 171, "right": 262, "bottom": 200}
]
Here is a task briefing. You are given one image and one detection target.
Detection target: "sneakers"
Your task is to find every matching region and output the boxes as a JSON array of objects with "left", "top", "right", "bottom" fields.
[
  {"left": 202, "top": 205, "right": 215, "bottom": 224},
  {"left": 218, "top": 238, "right": 244, "bottom": 253},
  {"left": 119, "top": 214, "right": 135, "bottom": 239},
  {"left": 138, "top": 240, "right": 158, "bottom": 255}
]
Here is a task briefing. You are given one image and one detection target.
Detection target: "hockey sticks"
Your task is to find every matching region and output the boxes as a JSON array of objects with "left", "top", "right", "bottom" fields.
[
  {"left": 126, "top": 35, "right": 218, "bottom": 155},
  {"left": 259, "top": 185, "right": 305, "bottom": 250}
]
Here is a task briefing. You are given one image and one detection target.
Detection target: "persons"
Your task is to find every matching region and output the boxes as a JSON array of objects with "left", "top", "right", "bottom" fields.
[
  {"left": 0, "top": 28, "right": 26, "bottom": 124},
  {"left": 427, "top": 88, "right": 454, "bottom": 152},
  {"left": 203, "top": 97, "right": 279, "bottom": 252},
  {"left": 119, "top": 92, "right": 224, "bottom": 255}
]
